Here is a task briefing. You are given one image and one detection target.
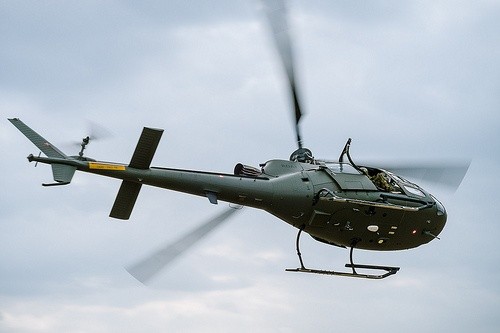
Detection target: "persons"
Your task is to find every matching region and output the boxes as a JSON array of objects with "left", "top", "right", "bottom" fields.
[{"left": 358, "top": 167, "right": 395, "bottom": 192}]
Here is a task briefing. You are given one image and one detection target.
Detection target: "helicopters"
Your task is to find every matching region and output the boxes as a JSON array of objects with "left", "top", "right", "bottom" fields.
[{"left": 1, "top": 1, "right": 447, "bottom": 279}]
